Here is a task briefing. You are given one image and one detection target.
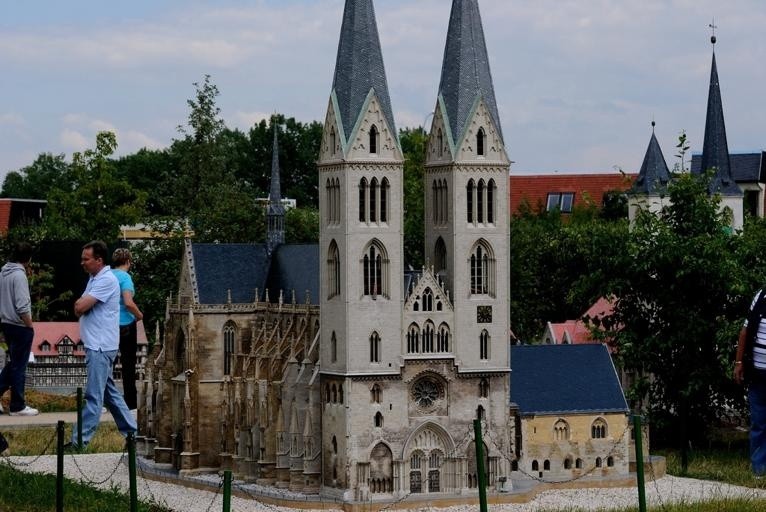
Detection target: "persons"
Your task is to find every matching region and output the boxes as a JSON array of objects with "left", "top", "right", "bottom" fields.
[
  {"left": 733, "top": 288, "right": 766, "bottom": 479},
  {"left": 64, "top": 240, "right": 145, "bottom": 451},
  {"left": 0, "top": 242, "right": 39, "bottom": 416}
]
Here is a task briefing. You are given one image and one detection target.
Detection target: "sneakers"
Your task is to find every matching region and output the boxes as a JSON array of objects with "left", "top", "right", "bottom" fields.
[{"left": 8, "top": 405, "right": 40, "bottom": 417}]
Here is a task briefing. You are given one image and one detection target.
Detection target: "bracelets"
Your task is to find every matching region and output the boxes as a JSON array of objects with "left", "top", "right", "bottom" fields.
[{"left": 734, "top": 361, "right": 743, "bottom": 365}]
